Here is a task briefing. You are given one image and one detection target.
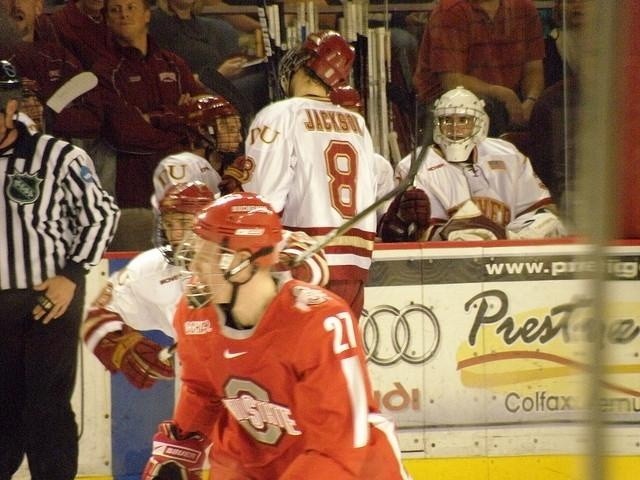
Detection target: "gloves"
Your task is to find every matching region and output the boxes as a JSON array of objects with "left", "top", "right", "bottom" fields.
[
  {"left": 94, "top": 324, "right": 174, "bottom": 390},
  {"left": 275, "top": 237, "right": 329, "bottom": 287},
  {"left": 379, "top": 187, "right": 431, "bottom": 241},
  {"left": 142, "top": 422, "right": 214, "bottom": 480},
  {"left": 329, "top": 86, "right": 360, "bottom": 106}
]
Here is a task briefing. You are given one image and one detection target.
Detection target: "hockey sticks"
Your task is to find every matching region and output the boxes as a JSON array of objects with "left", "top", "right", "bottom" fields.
[
  {"left": 377, "top": 132, "right": 418, "bottom": 236},
  {"left": 43, "top": 72, "right": 98, "bottom": 136},
  {"left": 158, "top": 111, "right": 436, "bottom": 362},
  {"left": 222, "top": 1, "right": 284, "bottom": 102}
]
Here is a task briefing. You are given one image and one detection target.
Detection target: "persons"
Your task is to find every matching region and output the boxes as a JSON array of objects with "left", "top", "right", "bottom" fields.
[{"left": 1, "top": 1, "right": 594, "bottom": 480}]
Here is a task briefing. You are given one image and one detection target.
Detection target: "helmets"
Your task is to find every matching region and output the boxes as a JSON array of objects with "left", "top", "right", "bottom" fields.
[
  {"left": 0, "top": 60, "right": 41, "bottom": 92},
  {"left": 151, "top": 151, "right": 220, "bottom": 216},
  {"left": 432, "top": 85, "right": 490, "bottom": 162},
  {"left": 190, "top": 191, "right": 283, "bottom": 264},
  {"left": 278, "top": 27, "right": 355, "bottom": 95},
  {"left": 187, "top": 95, "right": 239, "bottom": 127}
]
